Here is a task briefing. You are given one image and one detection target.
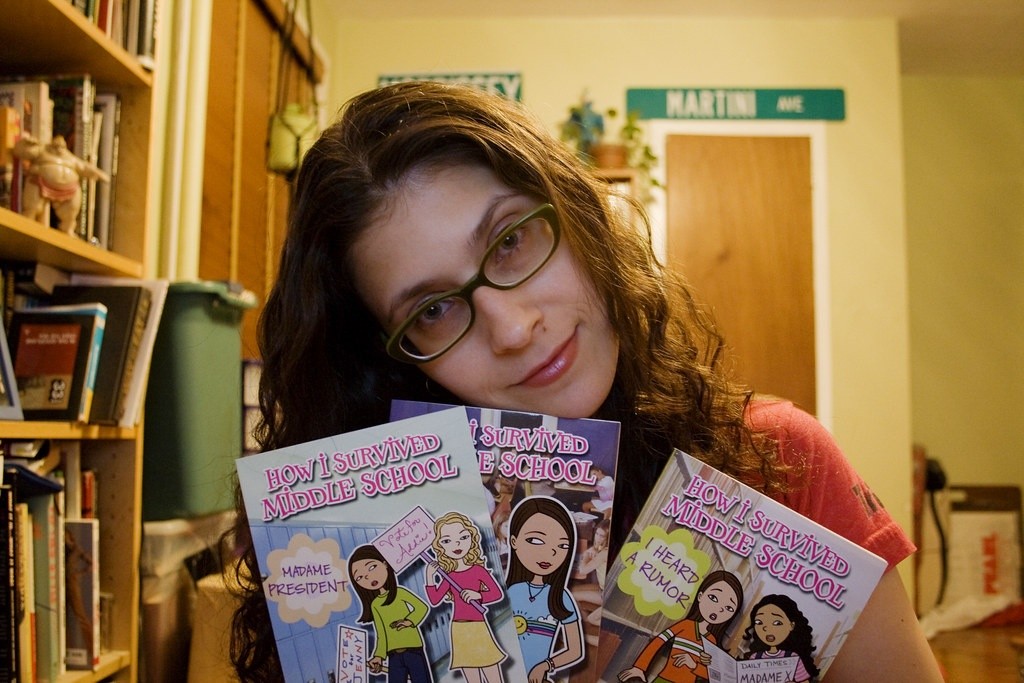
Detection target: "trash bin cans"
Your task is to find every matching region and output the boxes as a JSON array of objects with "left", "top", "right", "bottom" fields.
[{"left": 144, "top": 278, "right": 259, "bottom": 521}]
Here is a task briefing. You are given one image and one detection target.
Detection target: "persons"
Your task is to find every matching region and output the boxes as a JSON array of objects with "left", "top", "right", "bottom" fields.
[
  {"left": 13, "top": 133, "right": 112, "bottom": 236},
  {"left": 215, "top": 80, "right": 943, "bottom": 683}
]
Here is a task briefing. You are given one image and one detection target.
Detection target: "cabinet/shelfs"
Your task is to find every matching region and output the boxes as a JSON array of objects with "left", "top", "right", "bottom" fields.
[{"left": 0, "top": 0, "right": 178, "bottom": 682}]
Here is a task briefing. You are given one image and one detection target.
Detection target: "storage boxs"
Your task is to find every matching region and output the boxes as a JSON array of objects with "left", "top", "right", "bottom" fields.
[{"left": 143, "top": 281, "right": 261, "bottom": 522}]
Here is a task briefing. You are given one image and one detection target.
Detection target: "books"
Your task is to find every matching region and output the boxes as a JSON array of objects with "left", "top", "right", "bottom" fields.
[
  {"left": 0, "top": 1, "right": 168, "bottom": 683},
  {"left": 235, "top": 401, "right": 888, "bottom": 681}
]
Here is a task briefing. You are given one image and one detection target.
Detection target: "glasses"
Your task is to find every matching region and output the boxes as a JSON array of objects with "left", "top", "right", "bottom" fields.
[{"left": 386, "top": 200, "right": 562, "bottom": 364}]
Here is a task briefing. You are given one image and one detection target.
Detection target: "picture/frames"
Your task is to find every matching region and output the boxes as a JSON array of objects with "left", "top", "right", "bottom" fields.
[{"left": 7, "top": 310, "right": 96, "bottom": 419}]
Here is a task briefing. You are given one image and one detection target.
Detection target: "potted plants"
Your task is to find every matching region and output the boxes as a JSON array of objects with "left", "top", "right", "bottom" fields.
[{"left": 562, "top": 94, "right": 668, "bottom": 205}]
